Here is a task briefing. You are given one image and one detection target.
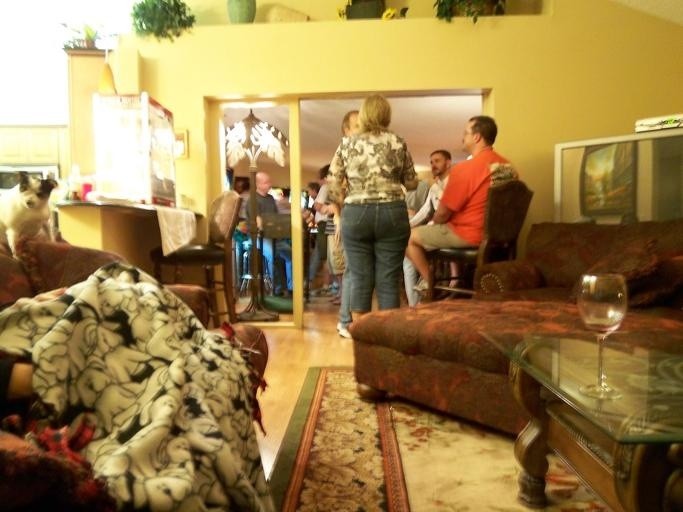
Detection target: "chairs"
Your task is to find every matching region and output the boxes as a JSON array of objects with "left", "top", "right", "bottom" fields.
[
  {"left": 414, "top": 177, "right": 535, "bottom": 304},
  {"left": 150, "top": 189, "right": 242, "bottom": 326}
]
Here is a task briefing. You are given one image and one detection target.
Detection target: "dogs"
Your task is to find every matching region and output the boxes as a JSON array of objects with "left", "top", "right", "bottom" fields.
[{"left": 0, "top": 167, "right": 57, "bottom": 262}]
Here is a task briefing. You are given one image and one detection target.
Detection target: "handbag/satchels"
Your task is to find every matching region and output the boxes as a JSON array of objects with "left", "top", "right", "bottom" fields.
[{"left": 325, "top": 235, "right": 345, "bottom": 275}]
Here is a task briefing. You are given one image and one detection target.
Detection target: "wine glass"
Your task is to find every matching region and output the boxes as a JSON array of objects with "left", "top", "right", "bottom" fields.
[{"left": 575, "top": 273, "right": 628, "bottom": 403}]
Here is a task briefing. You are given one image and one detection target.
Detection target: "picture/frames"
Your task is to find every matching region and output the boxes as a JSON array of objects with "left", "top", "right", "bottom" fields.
[{"left": 175, "top": 130, "right": 189, "bottom": 162}]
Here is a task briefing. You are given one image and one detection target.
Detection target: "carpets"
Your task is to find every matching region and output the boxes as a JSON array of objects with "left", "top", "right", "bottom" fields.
[{"left": 265, "top": 364, "right": 620, "bottom": 511}]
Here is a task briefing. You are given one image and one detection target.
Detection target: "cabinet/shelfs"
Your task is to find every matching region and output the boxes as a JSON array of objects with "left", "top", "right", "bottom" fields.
[{"left": 91, "top": 90, "right": 177, "bottom": 206}]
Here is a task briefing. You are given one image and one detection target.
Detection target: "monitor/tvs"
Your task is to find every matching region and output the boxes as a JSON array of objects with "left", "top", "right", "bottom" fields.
[{"left": 554, "top": 128, "right": 683, "bottom": 226}]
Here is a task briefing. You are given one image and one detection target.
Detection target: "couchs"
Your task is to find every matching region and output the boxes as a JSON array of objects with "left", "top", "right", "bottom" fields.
[
  {"left": 0, "top": 240, "right": 270, "bottom": 510},
  {"left": 349, "top": 220, "right": 682, "bottom": 451}
]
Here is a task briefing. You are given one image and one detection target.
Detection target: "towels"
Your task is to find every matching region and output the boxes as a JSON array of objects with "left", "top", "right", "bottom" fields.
[{"left": 155, "top": 204, "right": 195, "bottom": 257}]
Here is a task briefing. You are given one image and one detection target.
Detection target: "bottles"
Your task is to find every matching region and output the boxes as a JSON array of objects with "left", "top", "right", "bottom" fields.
[{"left": 64, "top": 181, "right": 93, "bottom": 203}]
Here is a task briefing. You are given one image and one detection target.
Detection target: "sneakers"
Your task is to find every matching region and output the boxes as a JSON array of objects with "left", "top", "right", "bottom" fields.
[
  {"left": 336, "top": 320, "right": 352, "bottom": 339},
  {"left": 412, "top": 276, "right": 429, "bottom": 291}
]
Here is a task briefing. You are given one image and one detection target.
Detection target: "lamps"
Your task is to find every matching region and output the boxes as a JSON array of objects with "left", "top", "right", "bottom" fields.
[
  {"left": 224, "top": 107, "right": 291, "bottom": 321},
  {"left": 93, "top": 35, "right": 117, "bottom": 96}
]
